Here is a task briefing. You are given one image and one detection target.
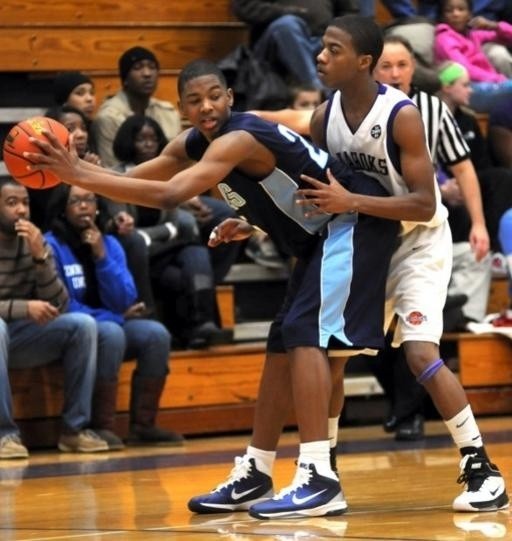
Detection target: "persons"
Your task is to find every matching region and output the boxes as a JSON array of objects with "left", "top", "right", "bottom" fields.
[
  {"left": 374, "top": 37, "right": 489, "bottom": 438},
  {"left": 1, "top": 46, "right": 237, "bottom": 457},
  {"left": 208, "top": 15, "right": 509, "bottom": 512},
  {"left": 230, "top": 1, "right": 512, "bottom": 322},
  {"left": 24, "top": 59, "right": 401, "bottom": 520}
]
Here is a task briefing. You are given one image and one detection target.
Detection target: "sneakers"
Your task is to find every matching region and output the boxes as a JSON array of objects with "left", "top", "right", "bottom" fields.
[
  {"left": 186, "top": 322, "right": 236, "bottom": 349},
  {"left": 491, "top": 310, "right": 512, "bottom": 337},
  {"left": 128, "top": 423, "right": 185, "bottom": 447},
  {"left": 247, "top": 460, "right": 349, "bottom": 519},
  {"left": 384, "top": 414, "right": 397, "bottom": 431},
  {"left": 186, "top": 458, "right": 278, "bottom": 514},
  {"left": 0, "top": 434, "right": 31, "bottom": 460},
  {"left": 396, "top": 413, "right": 427, "bottom": 442},
  {"left": 97, "top": 425, "right": 126, "bottom": 451},
  {"left": 57, "top": 429, "right": 110, "bottom": 453},
  {"left": 450, "top": 455, "right": 510, "bottom": 514}
]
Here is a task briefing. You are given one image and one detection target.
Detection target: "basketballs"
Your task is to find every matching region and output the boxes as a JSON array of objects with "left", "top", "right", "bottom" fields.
[{"left": 3, "top": 115, "right": 70, "bottom": 189}]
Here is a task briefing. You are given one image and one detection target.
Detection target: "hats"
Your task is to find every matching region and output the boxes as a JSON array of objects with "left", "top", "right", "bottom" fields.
[
  {"left": 49, "top": 70, "right": 93, "bottom": 105},
  {"left": 117, "top": 46, "right": 160, "bottom": 80}
]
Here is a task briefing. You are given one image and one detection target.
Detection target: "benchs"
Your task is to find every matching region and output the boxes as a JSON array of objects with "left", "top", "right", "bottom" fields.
[
  {"left": 209, "top": 283, "right": 235, "bottom": 341},
  {"left": 8, "top": 334, "right": 512, "bottom": 448}
]
